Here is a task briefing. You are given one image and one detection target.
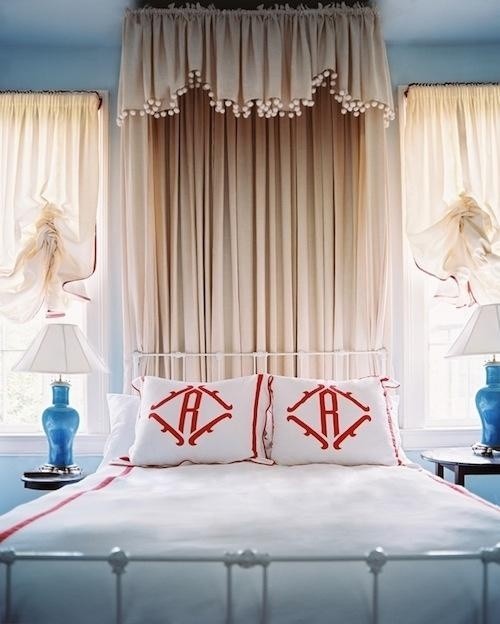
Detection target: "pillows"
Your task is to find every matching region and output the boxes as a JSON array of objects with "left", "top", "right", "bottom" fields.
[
  {"left": 96, "top": 394, "right": 141, "bottom": 469},
  {"left": 270, "top": 373, "right": 409, "bottom": 467},
  {"left": 109, "top": 372, "right": 274, "bottom": 467}
]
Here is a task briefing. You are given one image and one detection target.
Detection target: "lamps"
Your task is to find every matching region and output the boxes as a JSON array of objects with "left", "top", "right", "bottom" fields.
[
  {"left": 443, "top": 304, "right": 500, "bottom": 457},
  {"left": 11, "top": 323, "right": 107, "bottom": 476}
]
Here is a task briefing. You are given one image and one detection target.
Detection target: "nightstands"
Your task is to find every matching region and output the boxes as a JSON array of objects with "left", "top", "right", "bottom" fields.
[
  {"left": 20, "top": 473, "right": 86, "bottom": 491},
  {"left": 420, "top": 446, "right": 500, "bottom": 487}
]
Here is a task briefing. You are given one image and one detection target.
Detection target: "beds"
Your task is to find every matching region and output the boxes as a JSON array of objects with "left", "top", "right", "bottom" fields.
[{"left": 1, "top": 348, "right": 500, "bottom": 624}]
